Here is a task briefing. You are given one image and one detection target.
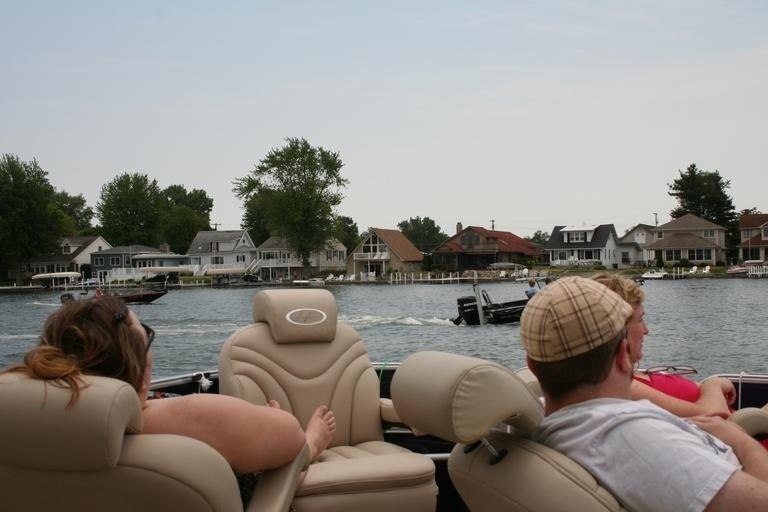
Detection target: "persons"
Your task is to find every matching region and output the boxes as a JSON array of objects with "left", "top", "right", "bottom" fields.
[
  {"left": 524, "top": 279, "right": 539, "bottom": 300},
  {"left": 592, "top": 274, "right": 767, "bottom": 450},
  {"left": 518, "top": 276, "right": 767, "bottom": 512},
  {"left": 95, "top": 287, "right": 103, "bottom": 300},
  {"left": 0, "top": 287, "right": 337, "bottom": 512}
]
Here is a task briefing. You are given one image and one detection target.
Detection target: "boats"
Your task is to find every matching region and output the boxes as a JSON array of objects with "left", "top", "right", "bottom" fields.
[
  {"left": 726, "top": 258, "right": 768, "bottom": 276},
  {"left": 449, "top": 273, "right": 553, "bottom": 331},
  {"left": 86, "top": 283, "right": 170, "bottom": 305},
  {"left": 641, "top": 269, "right": 669, "bottom": 279}
]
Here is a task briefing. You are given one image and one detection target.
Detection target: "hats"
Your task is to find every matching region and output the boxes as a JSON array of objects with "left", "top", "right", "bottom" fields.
[{"left": 520, "top": 275, "right": 634, "bottom": 363}]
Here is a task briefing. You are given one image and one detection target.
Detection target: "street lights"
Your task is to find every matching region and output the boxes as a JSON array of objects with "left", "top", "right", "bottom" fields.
[{"left": 651, "top": 211, "right": 660, "bottom": 269}]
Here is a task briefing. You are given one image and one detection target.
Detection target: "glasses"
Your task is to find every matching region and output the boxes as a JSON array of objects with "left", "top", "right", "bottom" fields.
[
  {"left": 140, "top": 321, "right": 156, "bottom": 353},
  {"left": 645, "top": 366, "right": 696, "bottom": 375}
]
